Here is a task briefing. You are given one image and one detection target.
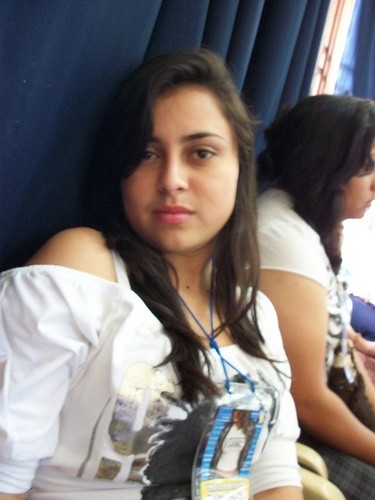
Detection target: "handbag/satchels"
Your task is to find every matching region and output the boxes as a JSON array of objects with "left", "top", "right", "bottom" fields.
[{"left": 329, "top": 339, "right": 375, "bottom": 433}]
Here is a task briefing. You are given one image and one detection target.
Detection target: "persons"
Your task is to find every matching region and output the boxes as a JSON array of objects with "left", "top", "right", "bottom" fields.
[
  {"left": 1, "top": 48, "right": 302, "bottom": 500},
  {"left": 249, "top": 95, "right": 375, "bottom": 500}
]
